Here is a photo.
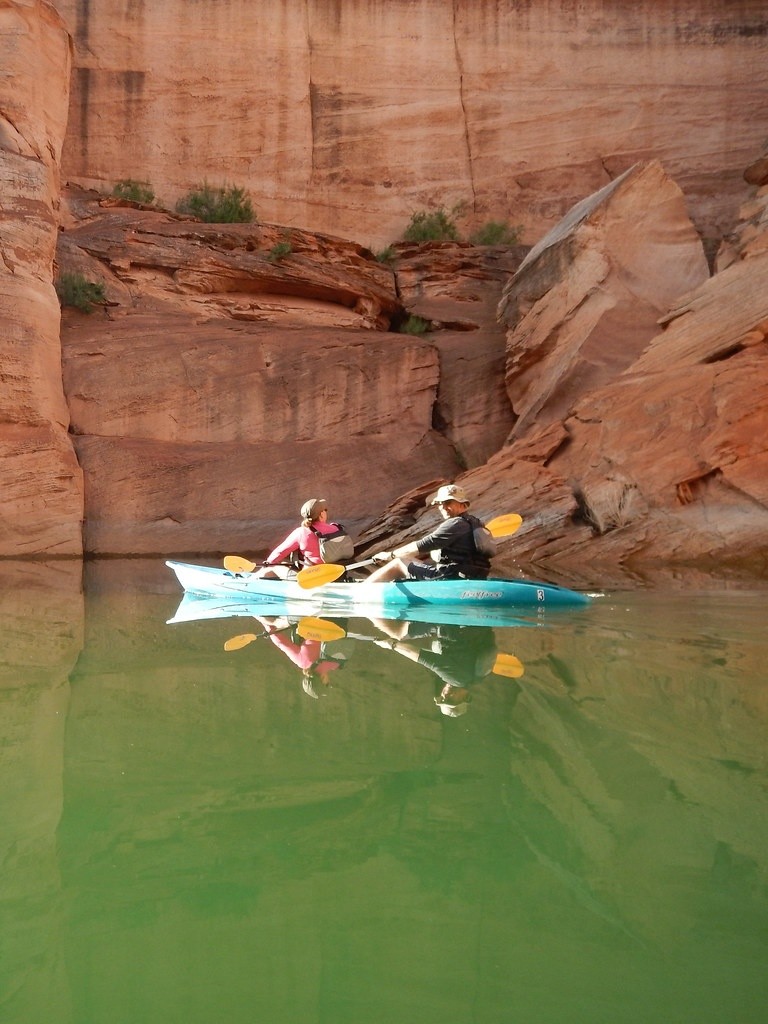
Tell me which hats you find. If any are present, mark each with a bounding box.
[301,499,328,521]
[433,696,469,717]
[302,675,327,699]
[431,485,470,507]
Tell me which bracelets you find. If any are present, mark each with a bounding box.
[391,550,395,559]
[391,641,399,653]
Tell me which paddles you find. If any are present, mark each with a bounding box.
[223,624,297,653]
[296,614,526,679]
[223,555,295,573]
[295,513,523,590]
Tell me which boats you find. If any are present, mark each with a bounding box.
[165,560,593,608]
[166,591,542,627]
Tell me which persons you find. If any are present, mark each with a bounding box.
[348,484,496,583]
[252,616,348,700]
[253,499,354,582]
[369,617,497,718]
